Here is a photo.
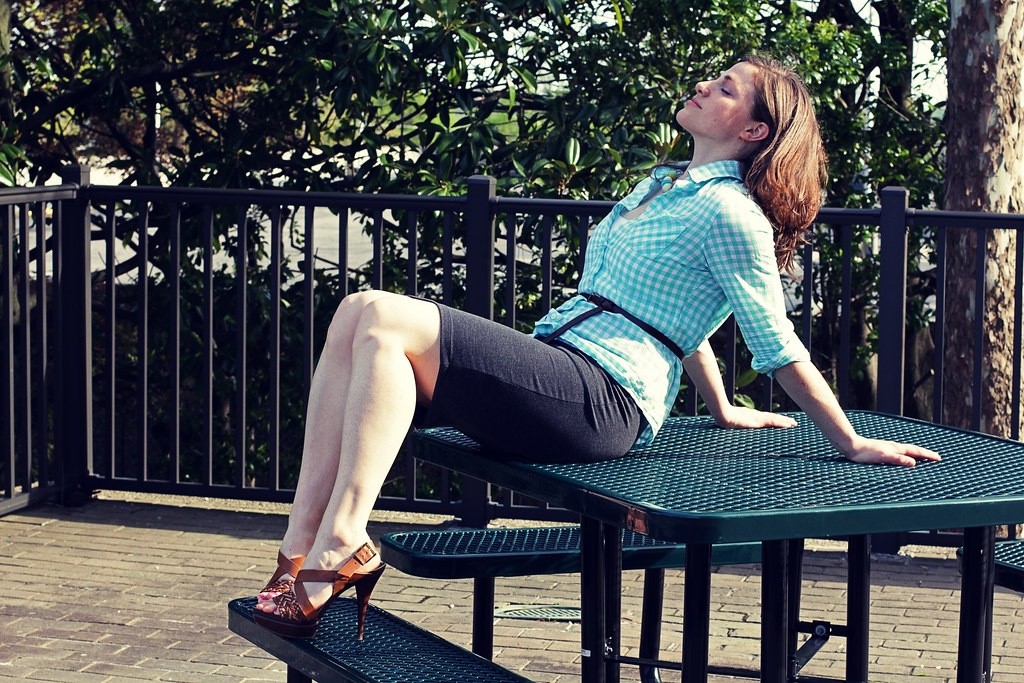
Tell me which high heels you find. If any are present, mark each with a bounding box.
[253,540,386,640]
[259,548,306,593]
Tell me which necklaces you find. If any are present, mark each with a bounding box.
[661,171,677,191]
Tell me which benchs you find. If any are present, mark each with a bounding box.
[227,596,533,683]
[956,540,1024,594]
[380,530,762,683]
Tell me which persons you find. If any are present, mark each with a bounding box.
[254,57,942,640]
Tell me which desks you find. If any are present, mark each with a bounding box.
[398,407,1024,683]
